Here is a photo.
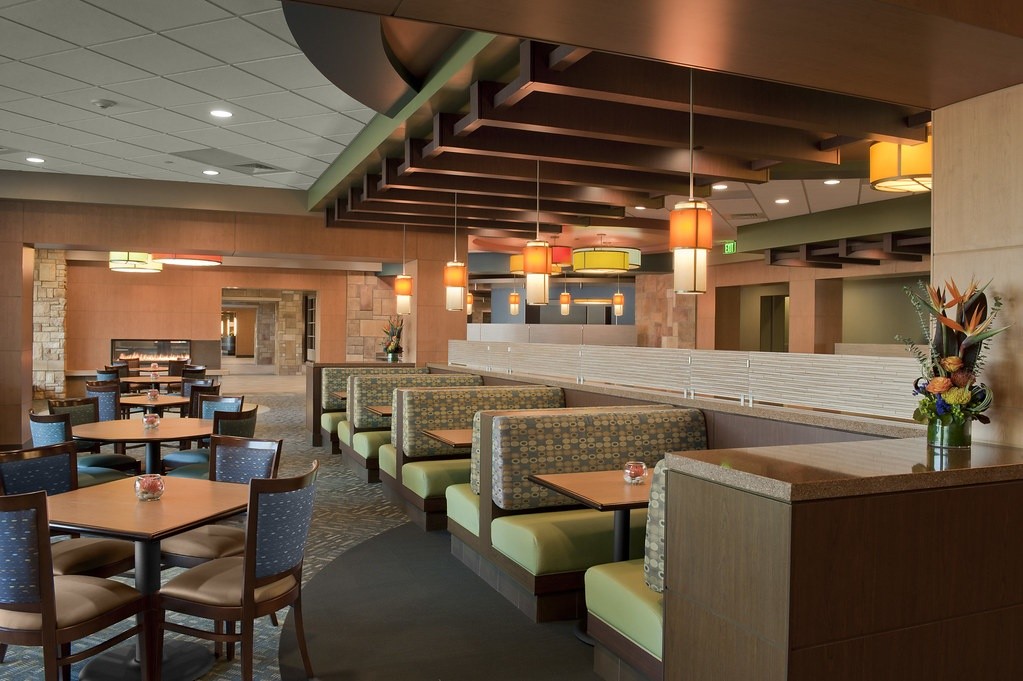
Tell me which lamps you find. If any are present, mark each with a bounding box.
[509,160,641,316]
[869,122,934,194]
[467,293,473,314]
[108,251,223,273]
[442,191,467,313]
[394,222,412,316]
[670,70,712,295]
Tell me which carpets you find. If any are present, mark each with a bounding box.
[278,520,654,681]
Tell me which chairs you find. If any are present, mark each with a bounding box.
[0,359,317,680]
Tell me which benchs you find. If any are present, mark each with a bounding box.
[319,366,711,681]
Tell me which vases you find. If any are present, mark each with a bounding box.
[387,350,399,363]
[924,410,971,448]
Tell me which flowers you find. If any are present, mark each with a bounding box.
[894,271,1015,422]
[379,316,404,351]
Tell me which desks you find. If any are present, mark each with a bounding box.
[420,428,474,448]
[364,406,393,417]
[119,374,184,392]
[128,365,167,377]
[47,474,258,681]
[70,418,214,477]
[118,394,193,417]
[329,390,346,401]
[527,467,655,647]
